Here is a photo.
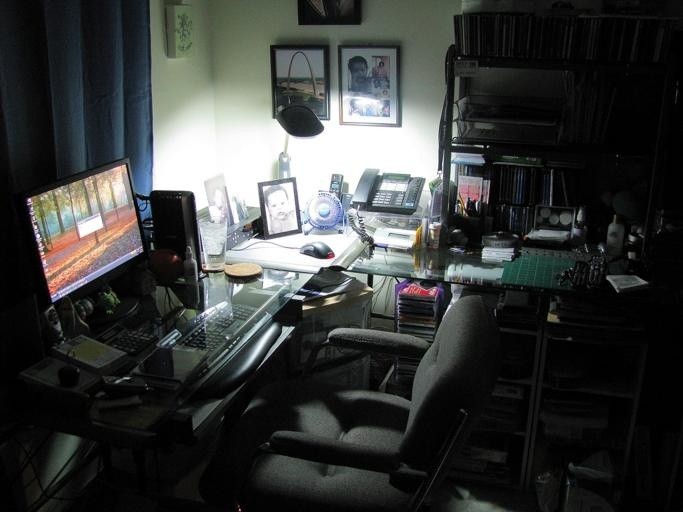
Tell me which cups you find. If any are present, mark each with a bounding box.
[198,215,228,272]
[627,232,645,261]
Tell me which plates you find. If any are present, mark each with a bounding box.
[224,262,262,277]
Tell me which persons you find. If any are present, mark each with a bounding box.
[262,184,297,234]
[346,56,372,95]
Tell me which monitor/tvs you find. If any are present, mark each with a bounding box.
[13,156,151,327]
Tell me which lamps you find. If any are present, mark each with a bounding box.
[276,105,323,178]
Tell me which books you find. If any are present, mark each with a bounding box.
[452,160,569,240]
[605,273,650,294]
[568,76,627,149]
[16,355,99,393]
[50,333,128,374]
[127,341,210,391]
[540,295,632,489]
[449,288,539,487]
[452,8,681,71]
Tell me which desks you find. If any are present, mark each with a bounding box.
[1,247,434,512]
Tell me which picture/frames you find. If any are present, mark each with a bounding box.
[257,178,305,240]
[268,43,406,132]
[298,0,363,26]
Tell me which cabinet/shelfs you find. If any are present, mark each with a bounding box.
[435,0,682,512]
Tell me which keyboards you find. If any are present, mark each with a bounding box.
[133,273,273,378]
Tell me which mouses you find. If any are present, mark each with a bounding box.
[299,239,336,259]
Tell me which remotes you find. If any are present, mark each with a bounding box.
[588,254,608,290]
[571,260,588,289]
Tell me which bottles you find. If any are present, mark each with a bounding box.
[573,207,588,247]
[606,213,626,255]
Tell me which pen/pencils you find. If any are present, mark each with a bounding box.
[454,192,483,217]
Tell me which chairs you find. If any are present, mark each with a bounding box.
[250,293,500,512]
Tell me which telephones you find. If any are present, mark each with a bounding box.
[350,168,425,215]
